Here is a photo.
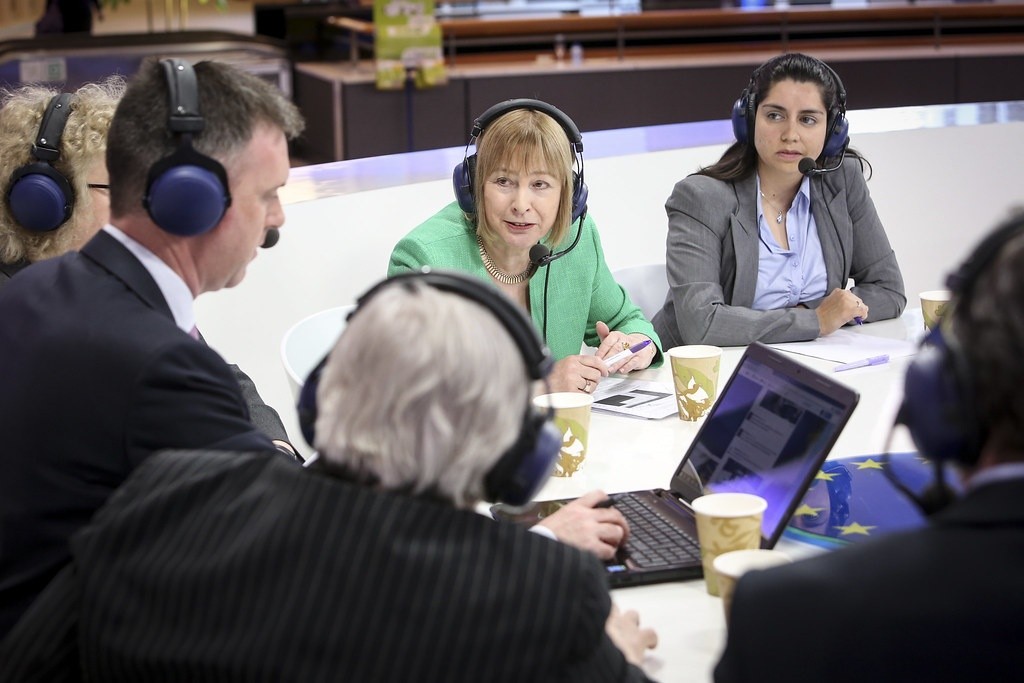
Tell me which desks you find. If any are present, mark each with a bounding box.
[474,307,959,683]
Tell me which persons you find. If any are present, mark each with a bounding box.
[0,57,629,629]
[0,269,665,683]
[0,76,128,284]
[649,52,909,350]
[386,98,665,398]
[715,212,1024,683]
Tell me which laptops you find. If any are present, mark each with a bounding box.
[489,341,859,590]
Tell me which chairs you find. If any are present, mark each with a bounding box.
[279,303,356,407]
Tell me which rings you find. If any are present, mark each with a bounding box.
[583,380,591,392]
[622,342,629,350]
[856,301,860,307]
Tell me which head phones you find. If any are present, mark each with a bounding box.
[4,94,76,235]
[732,55,850,158]
[295,269,560,509]
[903,219,1024,464]
[452,99,588,225]
[140,57,233,237]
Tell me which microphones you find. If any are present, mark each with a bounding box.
[530,217,585,266]
[798,151,845,176]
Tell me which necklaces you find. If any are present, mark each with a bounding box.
[760,191,789,223]
[474,225,534,284]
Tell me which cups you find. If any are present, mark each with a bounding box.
[920,291,951,339]
[692,494,768,597]
[714,549,790,630]
[667,345,721,424]
[532,393,593,477]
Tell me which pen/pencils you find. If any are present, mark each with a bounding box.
[855,317,862,325]
[603,339,652,369]
[831,354,890,372]
[594,489,630,510]
